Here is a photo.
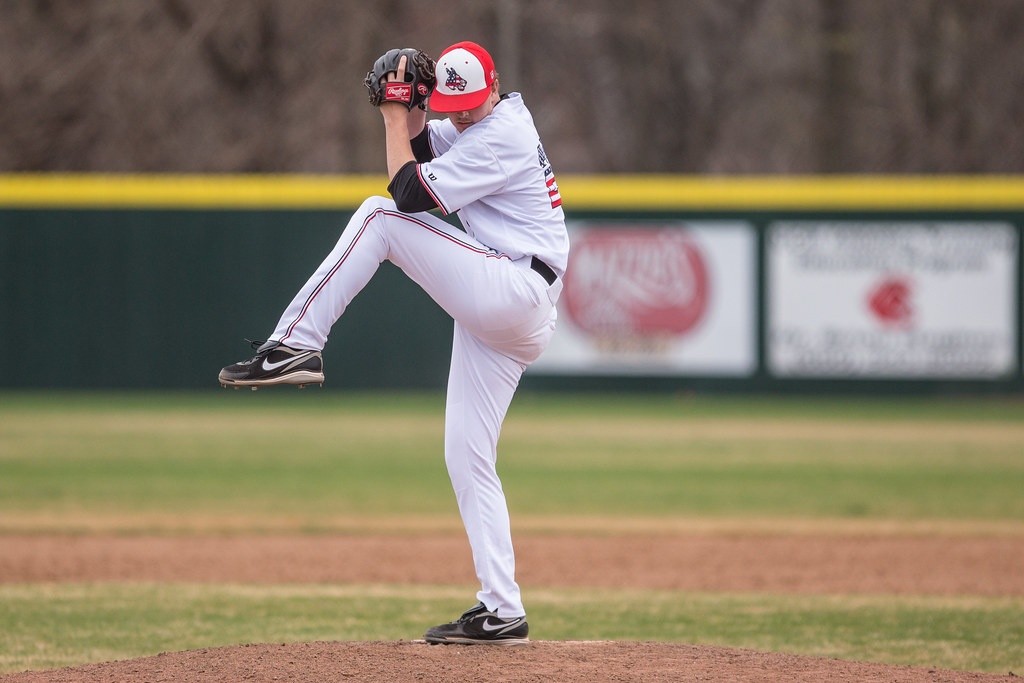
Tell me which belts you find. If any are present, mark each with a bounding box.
[530,256,557,286]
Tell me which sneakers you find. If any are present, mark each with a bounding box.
[218,338,324,391]
[423,602,529,645]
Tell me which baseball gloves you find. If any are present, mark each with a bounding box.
[365,48,436,113]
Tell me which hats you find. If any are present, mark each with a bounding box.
[429,41,496,112]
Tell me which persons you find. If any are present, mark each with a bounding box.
[218,41,569,645]
